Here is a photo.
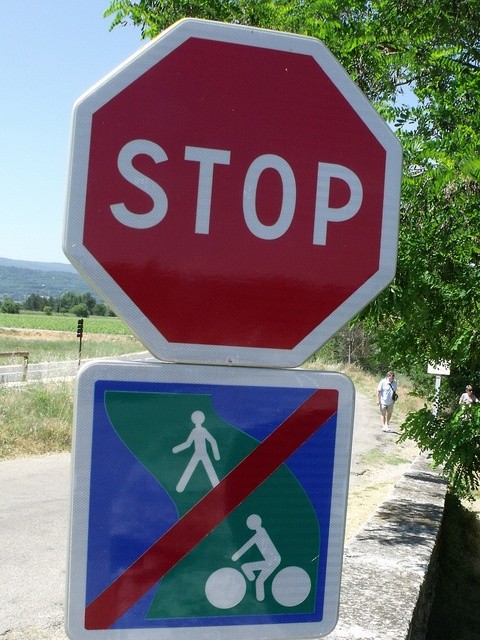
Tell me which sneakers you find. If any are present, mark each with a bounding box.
[382,426,386,431]
[386,425,391,430]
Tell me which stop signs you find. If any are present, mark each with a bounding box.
[65,362,355,639]
[63,17,404,369]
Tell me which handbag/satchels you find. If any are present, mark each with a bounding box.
[389,384,398,400]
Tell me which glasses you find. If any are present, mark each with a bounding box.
[467,389,473,392]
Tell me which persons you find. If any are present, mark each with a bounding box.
[375,370,399,433]
[458,384,479,419]
